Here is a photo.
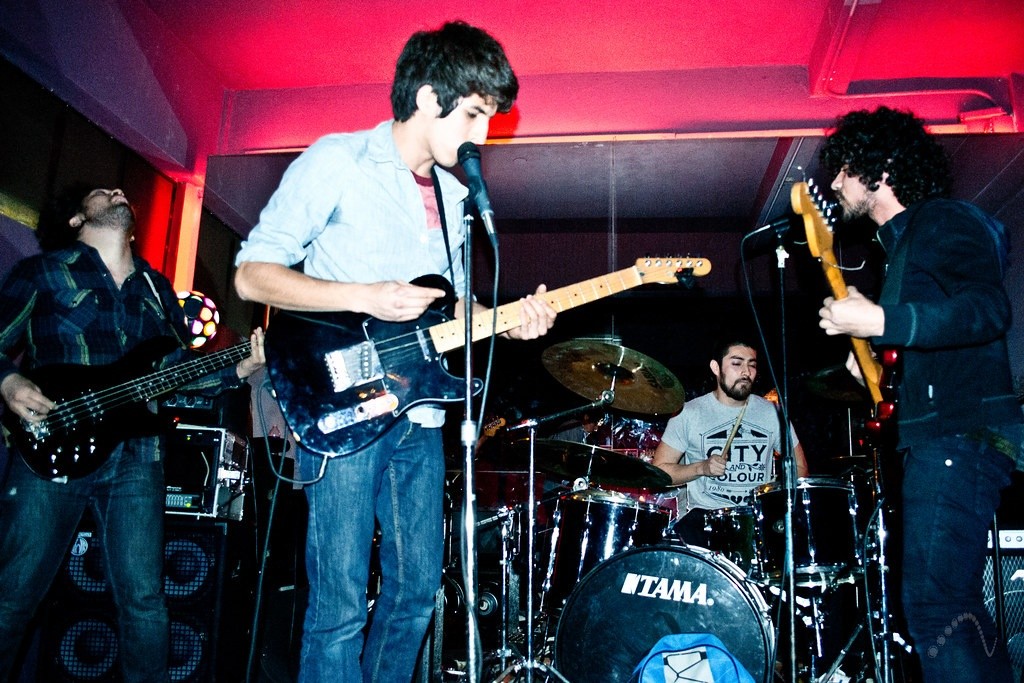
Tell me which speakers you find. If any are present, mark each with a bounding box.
[20,515,252,683]
[981,548,1024,683]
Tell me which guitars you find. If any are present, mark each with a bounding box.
[264,253,713,460]
[787,178,901,467]
[0,336,253,484]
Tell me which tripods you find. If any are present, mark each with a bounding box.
[822,461,921,683]
[476,376,615,683]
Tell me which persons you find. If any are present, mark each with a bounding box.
[0,178,266,683]
[234,20,558,683]
[818,106,1023,683]
[541,400,610,494]
[652,336,810,683]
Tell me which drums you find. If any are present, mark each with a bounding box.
[750,476,863,588]
[540,489,674,613]
[703,504,785,588]
[551,544,779,683]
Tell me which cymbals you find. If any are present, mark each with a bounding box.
[545,340,688,419]
[508,434,675,498]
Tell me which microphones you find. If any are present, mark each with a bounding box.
[458,141,499,248]
[744,201,843,239]
